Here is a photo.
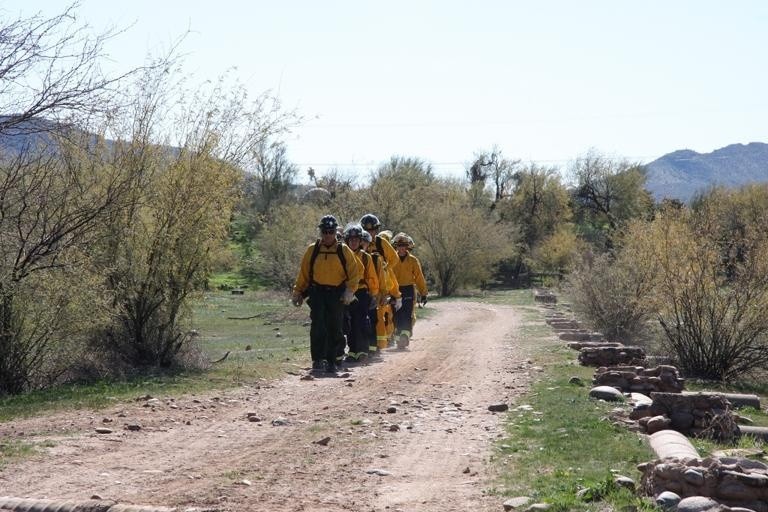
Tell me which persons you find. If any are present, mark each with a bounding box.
[292,213,428,375]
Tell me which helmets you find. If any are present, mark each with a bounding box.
[391,232,416,249]
[343,223,362,238]
[316,215,339,228]
[359,213,382,230]
[361,230,373,243]
[377,229,393,242]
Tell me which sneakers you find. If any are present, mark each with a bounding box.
[311,333,410,373]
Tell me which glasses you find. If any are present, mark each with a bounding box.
[321,229,336,235]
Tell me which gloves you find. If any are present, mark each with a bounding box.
[291,293,306,308]
[394,298,403,312]
[339,287,355,306]
[369,296,378,311]
[420,295,428,307]
